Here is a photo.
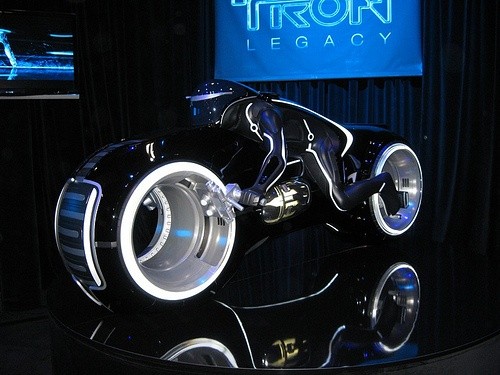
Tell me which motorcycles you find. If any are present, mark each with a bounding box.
[52,122,426,316]
[86,241,425,369]
